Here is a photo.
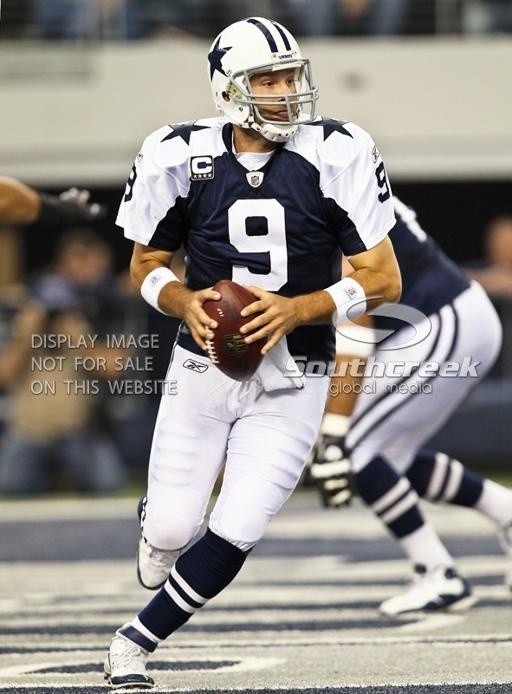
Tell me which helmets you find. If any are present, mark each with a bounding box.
[208,16,319,144]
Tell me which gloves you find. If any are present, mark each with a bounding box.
[310,432,355,509]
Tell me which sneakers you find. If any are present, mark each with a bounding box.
[102,627,156,689]
[136,496,183,591]
[500,521,512,593]
[377,562,479,622]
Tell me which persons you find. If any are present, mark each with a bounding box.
[308,194,511,621]
[454,202,511,381]
[103,17,403,688]
[0,174,182,499]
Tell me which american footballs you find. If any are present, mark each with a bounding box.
[202,280,268,382]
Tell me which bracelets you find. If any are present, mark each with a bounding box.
[323,276,367,326]
[139,266,181,316]
